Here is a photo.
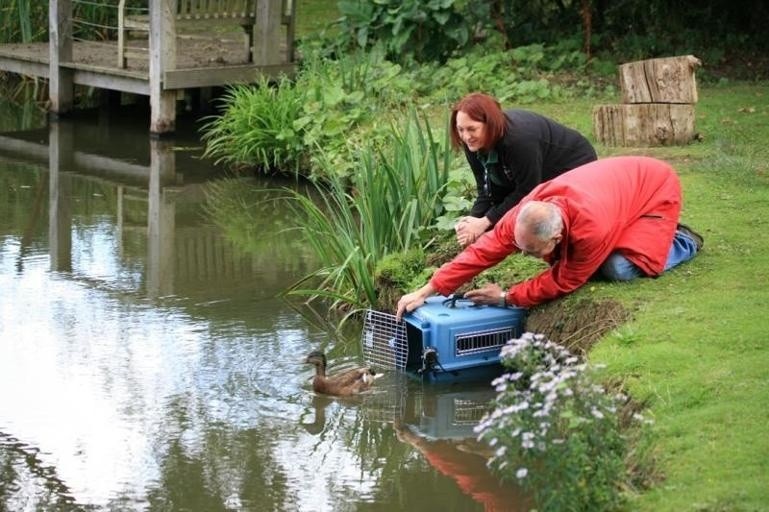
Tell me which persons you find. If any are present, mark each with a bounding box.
[449,93,598,248]
[396,156,705,325]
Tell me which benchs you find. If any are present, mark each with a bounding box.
[118,0,296,68]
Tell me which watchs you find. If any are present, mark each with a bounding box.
[497,289,507,309]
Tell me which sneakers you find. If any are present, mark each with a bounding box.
[675,222,704,250]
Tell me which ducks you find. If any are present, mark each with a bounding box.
[299,350,388,396]
